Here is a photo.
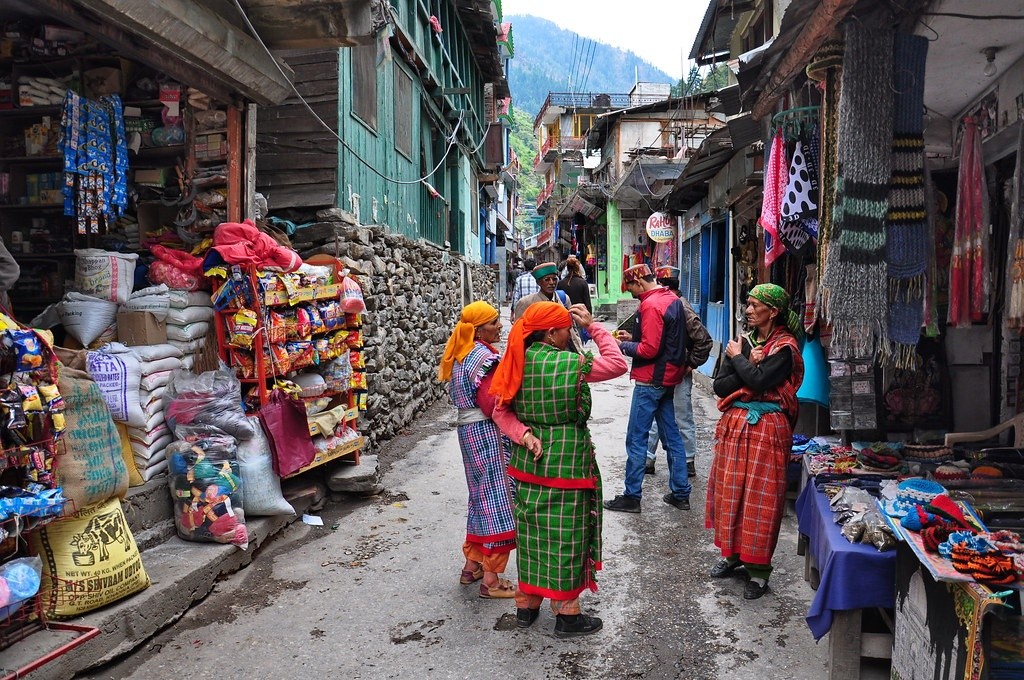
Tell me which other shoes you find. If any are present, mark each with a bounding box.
[711,559,744,577]
[744,579,768,599]
[686,461,696,477]
[645,460,655,474]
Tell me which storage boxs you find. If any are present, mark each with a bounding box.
[136,168,168,187]
[125,116,156,132]
[116,312,168,346]
[81,55,134,102]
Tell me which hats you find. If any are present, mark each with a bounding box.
[654,266,680,279]
[624,264,653,283]
[531,262,557,281]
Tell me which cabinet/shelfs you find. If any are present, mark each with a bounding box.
[0,48,239,318]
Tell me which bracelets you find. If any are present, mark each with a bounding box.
[523,431,531,439]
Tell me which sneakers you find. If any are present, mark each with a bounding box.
[663,493,690,510]
[604,494,641,513]
[517,608,539,627]
[479,577,517,598]
[460,563,484,584]
[554,613,602,637]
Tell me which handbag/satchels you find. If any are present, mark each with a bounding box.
[257,388,315,477]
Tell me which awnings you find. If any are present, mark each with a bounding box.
[664,114,761,216]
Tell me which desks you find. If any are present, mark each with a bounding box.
[794,452,898,680]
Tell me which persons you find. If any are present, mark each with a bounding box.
[513,262,579,339]
[557,253,592,316]
[703,283,805,599]
[486,301,630,638]
[436,300,520,600]
[510,258,542,325]
[599,264,691,512]
[0,236,21,311]
[644,264,713,476]
